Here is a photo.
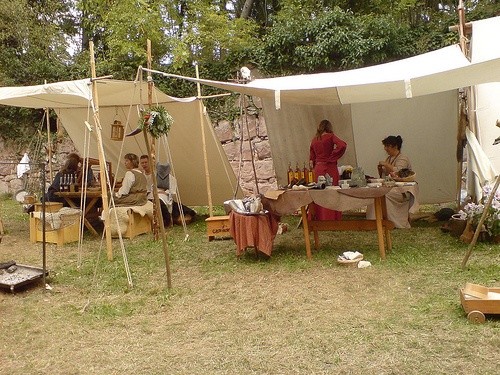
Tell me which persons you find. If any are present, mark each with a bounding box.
[139,154,177,229]
[307,120,347,221]
[99,153,147,225]
[47,153,98,222]
[367,135,417,229]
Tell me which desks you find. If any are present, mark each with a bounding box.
[54,188,119,237]
[263,180,417,260]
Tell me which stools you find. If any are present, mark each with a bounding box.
[30,201,411,248]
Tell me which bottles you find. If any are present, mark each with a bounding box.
[243,187,255,213]
[343,167,352,179]
[287,160,314,185]
[60,174,79,192]
[254,193,263,213]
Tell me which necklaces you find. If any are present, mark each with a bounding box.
[389,153,400,164]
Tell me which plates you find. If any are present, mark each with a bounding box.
[88,187,100,191]
[394,181,417,187]
[368,178,386,183]
[327,185,342,190]
[368,183,383,188]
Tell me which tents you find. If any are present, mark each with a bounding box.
[138,15,500,269]
[0,70,247,293]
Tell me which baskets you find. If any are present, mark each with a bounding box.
[391,168,416,182]
